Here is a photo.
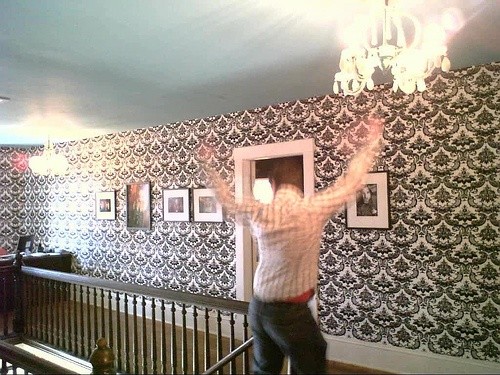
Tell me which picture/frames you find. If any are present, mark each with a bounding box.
[125,181,151,231]
[192,188,224,223]
[162,187,190,222]
[345,170,391,230]
[95,191,116,220]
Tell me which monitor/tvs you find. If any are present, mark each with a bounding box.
[17,235,35,255]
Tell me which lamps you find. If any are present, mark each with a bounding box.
[333,0,451,96]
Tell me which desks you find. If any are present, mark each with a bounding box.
[0,253,71,312]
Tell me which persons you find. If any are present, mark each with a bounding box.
[355,184,378,216]
[100,199,110,212]
[193,116,384,375]
